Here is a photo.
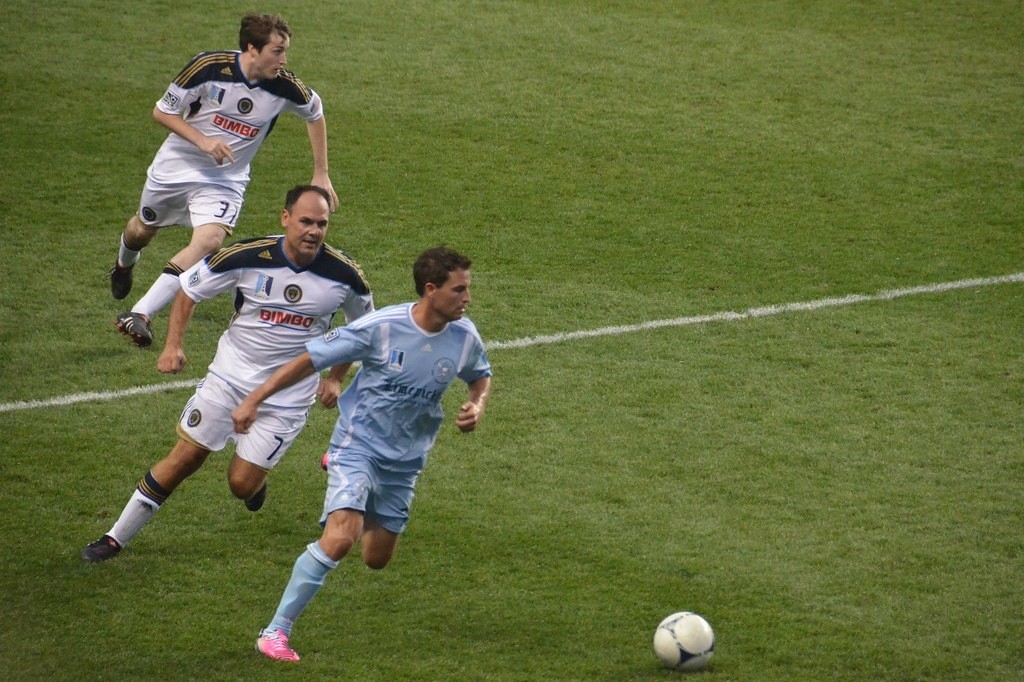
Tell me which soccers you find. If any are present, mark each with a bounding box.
[653,612,715,672]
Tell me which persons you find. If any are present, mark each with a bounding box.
[108,14,340,349]
[228,249,494,662]
[79,184,378,561]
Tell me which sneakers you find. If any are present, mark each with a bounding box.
[255,628,300,663]
[245,480,267,511]
[322,451,327,471]
[109,255,135,299]
[81,535,121,562]
[113,312,153,348]
